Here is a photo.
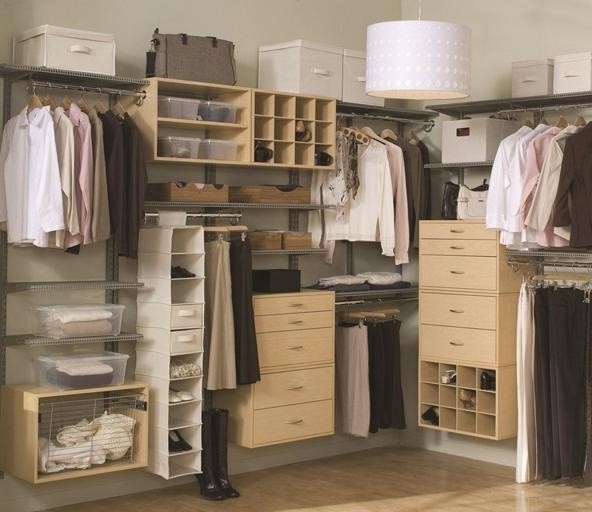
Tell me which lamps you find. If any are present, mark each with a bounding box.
[365,0,470,101]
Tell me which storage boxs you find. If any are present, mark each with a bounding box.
[157,134,203,160]
[510,59,553,96]
[157,95,202,121]
[32,349,129,392]
[24,303,125,339]
[198,139,240,162]
[198,100,240,123]
[256,39,342,102]
[10,24,115,77]
[440,118,522,164]
[342,48,384,108]
[552,53,592,97]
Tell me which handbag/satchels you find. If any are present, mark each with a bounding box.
[455,184,489,220]
[145,50,156,78]
[441,181,459,220]
[152,32,239,86]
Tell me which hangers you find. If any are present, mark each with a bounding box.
[333,298,400,320]
[521,103,586,129]
[519,261,592,303]
[222,213,250,243]
[21,81,127,119]
[198,217,228,243]
[336,111,422,148]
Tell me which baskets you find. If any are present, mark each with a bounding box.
[37,391,139,477]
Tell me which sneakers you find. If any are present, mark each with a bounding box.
[171,267,196,278]
[169,389,181,402]
[174,389,193,401]
[420,405,440,427]
[441,368,456,384]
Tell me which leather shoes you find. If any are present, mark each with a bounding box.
[481,371,496,392]
[172,429,192,450]
[170,434,182,451]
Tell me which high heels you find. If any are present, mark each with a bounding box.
[459,388,476,409]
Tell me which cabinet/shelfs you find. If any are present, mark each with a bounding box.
[251,88,337,171]
[139,201,328,257]
[3,280,144,352]
[419,219,538,367]
[210,288,337,451]
[416,359,518,440]
[0,379,150,485]
[135,225,203,481]
[115,77,252,167]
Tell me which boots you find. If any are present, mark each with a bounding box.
[209,407,240,497]
[195,412,226,500]
